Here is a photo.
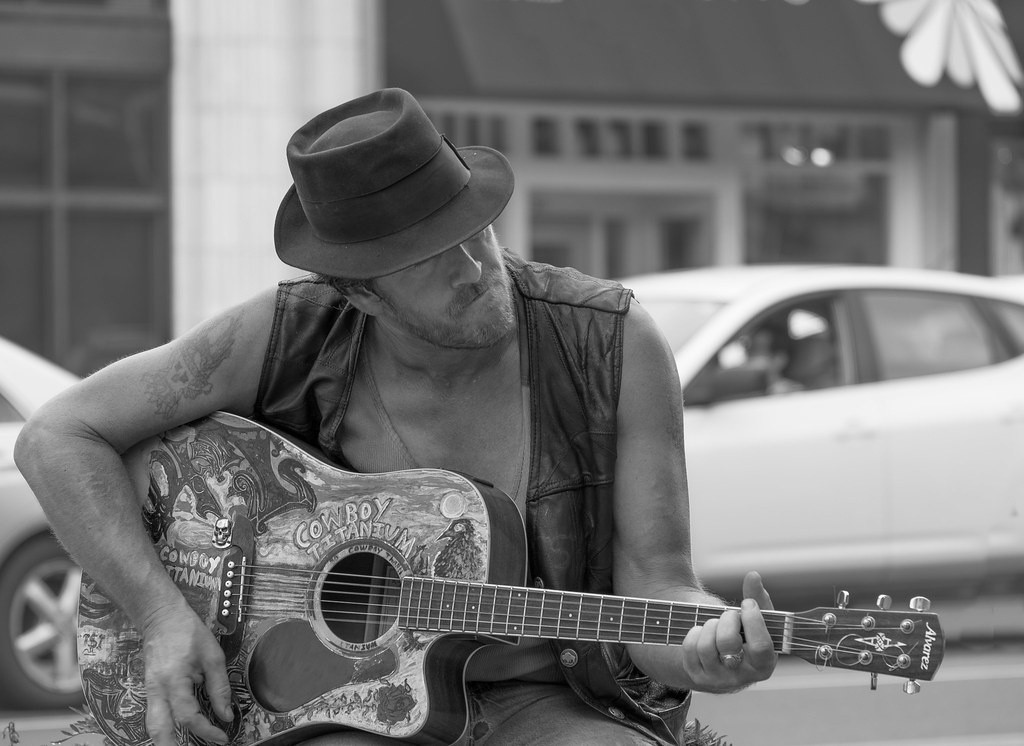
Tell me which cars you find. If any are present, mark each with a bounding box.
[582,260,1023,644]
[0,332,125,716]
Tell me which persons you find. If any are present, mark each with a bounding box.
[745,320,807,397]
[12,84,780,746]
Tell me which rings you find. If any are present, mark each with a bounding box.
[717,647,746,672]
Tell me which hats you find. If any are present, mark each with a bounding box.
[274,86,515,281]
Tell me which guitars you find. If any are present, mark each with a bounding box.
[74,409,947,746]
